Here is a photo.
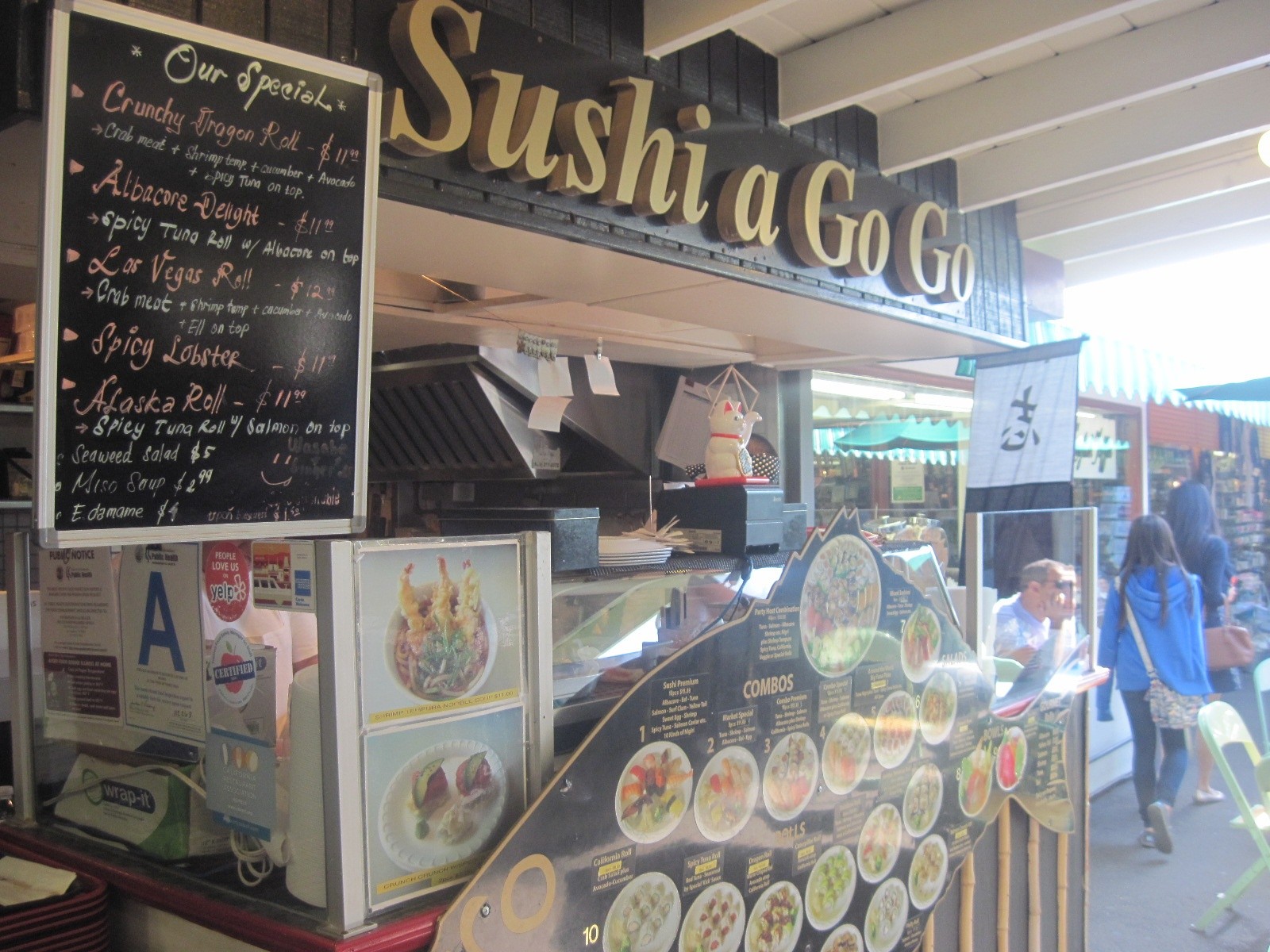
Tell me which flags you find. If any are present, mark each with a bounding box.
[966,339,1080,513]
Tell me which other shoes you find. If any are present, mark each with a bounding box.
[1148,802,1174,854]
[1195,788,1223,804]
[1143,828,1154,849]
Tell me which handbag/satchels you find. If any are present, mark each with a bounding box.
[1199,593,1255,669]
[1150,680,1205,729]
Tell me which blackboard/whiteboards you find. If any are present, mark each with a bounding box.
[31,0,383,553]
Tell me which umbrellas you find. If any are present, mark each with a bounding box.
[1173,377,1270,402]
[833,415,1130,458]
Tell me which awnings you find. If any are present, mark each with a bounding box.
[812,428,959,467]
[956,321,1270,426]
[811,372,1103,438]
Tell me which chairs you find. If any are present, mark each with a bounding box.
[1189,658,1270,931]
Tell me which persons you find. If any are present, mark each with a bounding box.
[1096,516,1214,854]
[996,515,1053,598]
[1164,480,1238,802]
[986,558,1066,665]
[1076,523,1119,578]
[1042,564,1076,660]
[203,536,318,718]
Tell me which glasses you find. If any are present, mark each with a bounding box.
[1042,579,1064,589]
